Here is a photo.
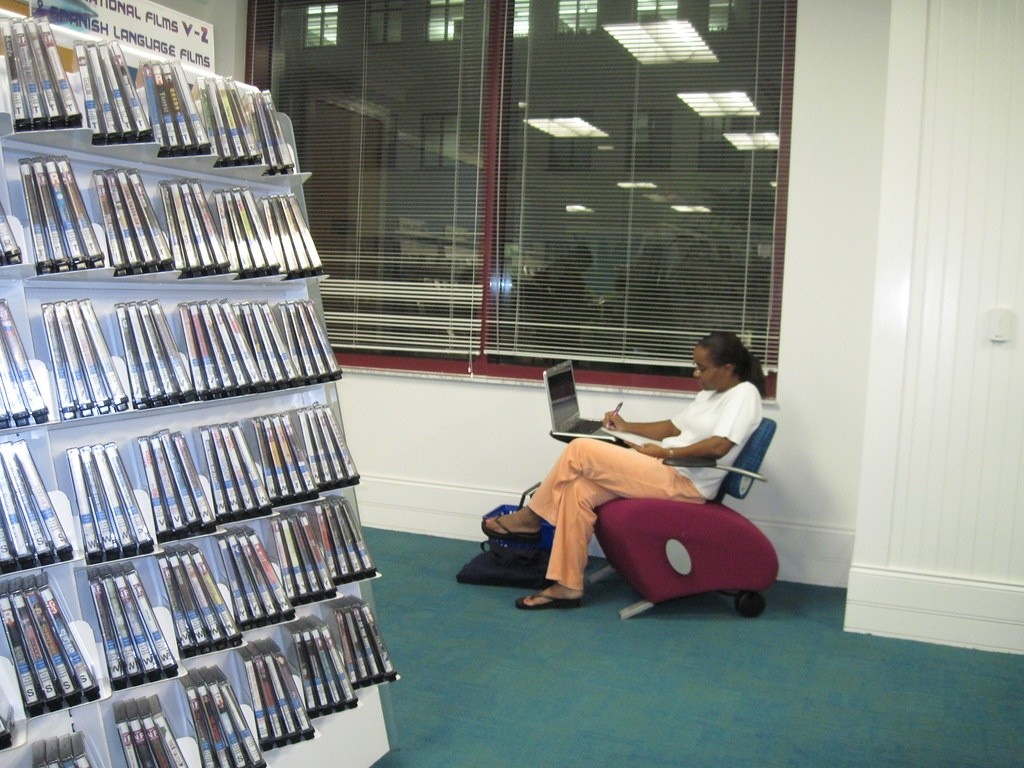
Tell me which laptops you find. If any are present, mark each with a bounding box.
[543,360,619,443]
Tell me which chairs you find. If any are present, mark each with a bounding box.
[582,417,779,624]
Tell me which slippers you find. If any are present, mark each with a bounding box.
[515,594,580,609]
[482,515,541,544]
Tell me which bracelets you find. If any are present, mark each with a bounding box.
[669,450,674,458]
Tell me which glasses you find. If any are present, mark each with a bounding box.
[692,360,724,374]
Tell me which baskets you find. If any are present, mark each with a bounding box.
[483,481,556,550]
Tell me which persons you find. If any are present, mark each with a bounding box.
[482,330,764,610]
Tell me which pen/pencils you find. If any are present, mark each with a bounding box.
[606,400,623,429]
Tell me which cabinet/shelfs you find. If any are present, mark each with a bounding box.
[0,11,393,768]
